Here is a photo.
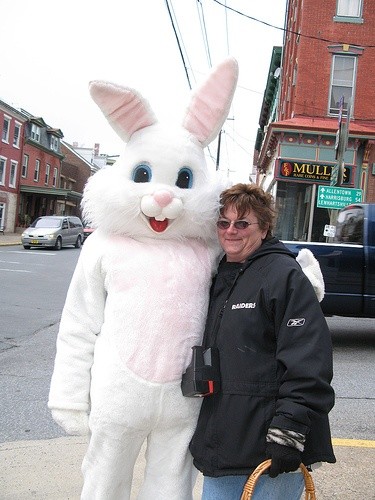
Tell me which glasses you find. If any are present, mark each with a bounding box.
[216,217,259,230]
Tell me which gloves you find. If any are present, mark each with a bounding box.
[51,409,89,436]
[266,441,301,478]
[296,248,325,303]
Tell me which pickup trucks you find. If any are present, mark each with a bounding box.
[271,205,375,320]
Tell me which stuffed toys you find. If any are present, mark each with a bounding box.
[48,56,325,500]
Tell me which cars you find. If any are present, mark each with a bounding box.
[20,215,84,251]
[83,224,94,241]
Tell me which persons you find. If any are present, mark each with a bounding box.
[189,183,336,500]
